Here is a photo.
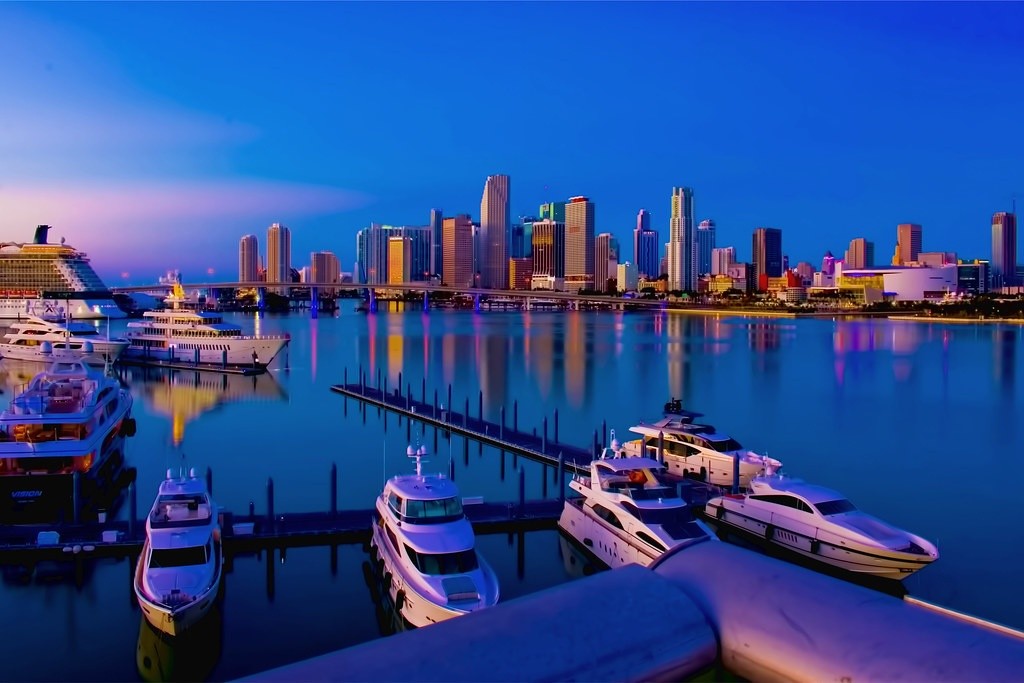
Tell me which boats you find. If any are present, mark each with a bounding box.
[370,436,500,631]
[0,291,40,299]
[133,464,225,640]
[137,597,224,683]
[1,295,132,365]
[557,428,720,569]
[704,450,939,583]
[620,397,784,492]
[0,300,136,525]
[120,276,292,369]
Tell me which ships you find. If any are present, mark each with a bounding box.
[0,225,128,320]
